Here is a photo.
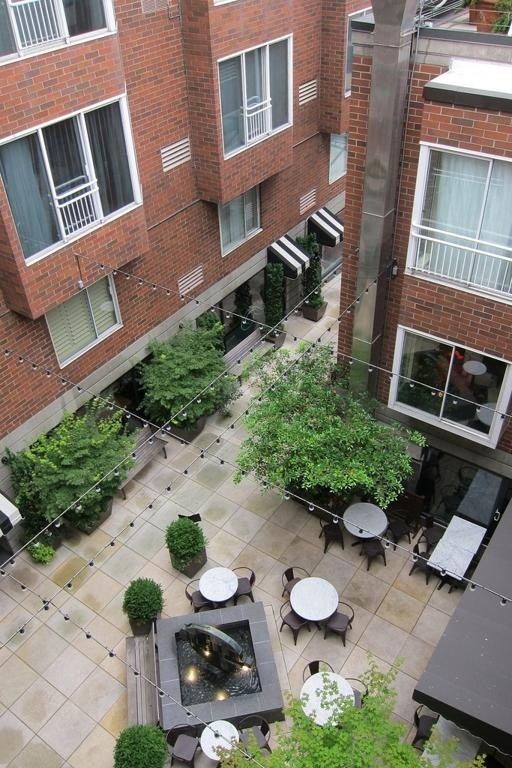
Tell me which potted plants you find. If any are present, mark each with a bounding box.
[260,262,288,348]
[138,348,227,444]
[14,394,136,535]
[166,516,209,578]
[120,578,164,638]
[298,232,329,322]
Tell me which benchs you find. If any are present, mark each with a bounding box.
[217,327,274,386]
[107,424,168,500]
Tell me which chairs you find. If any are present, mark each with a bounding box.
[183,567,256,611]
[301,659,369,719]
[318,508,412,570]
[279,567,355,647]
[163,715,271,768]
[409,466,505,592]
[411,705,440,751]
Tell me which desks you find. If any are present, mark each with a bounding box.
[298,672,355,729]
[344,502,389,546]
[420,715,486,768]
